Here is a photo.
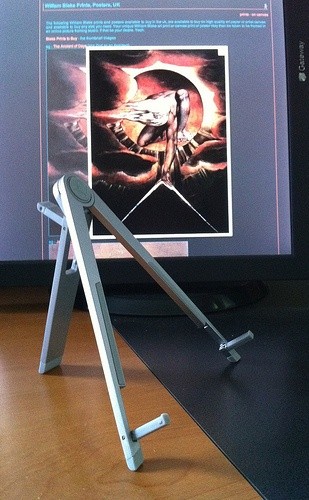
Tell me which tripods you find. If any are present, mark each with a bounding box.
[24,170,255,472]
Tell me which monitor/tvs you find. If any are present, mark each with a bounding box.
[0,1,308,287]
